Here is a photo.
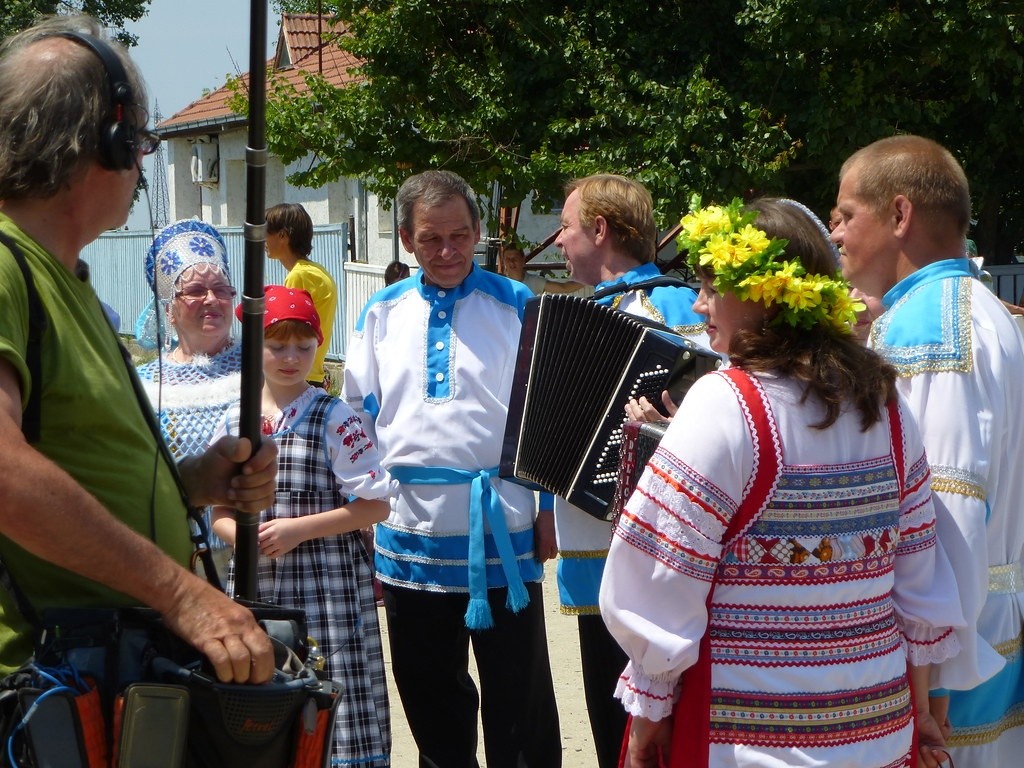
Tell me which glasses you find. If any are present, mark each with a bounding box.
[130,125,164,155]
[171,286,237,301]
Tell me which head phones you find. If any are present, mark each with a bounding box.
[56,31,138,169]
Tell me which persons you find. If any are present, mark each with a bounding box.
[338,170,731,768]
[384,260,411,287]
[0,10,393,767]
[504,242,585,296]
[599,133,1024,768]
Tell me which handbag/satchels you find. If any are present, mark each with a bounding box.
[1,598,344,768]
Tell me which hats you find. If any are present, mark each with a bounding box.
[142,219,231,350]
